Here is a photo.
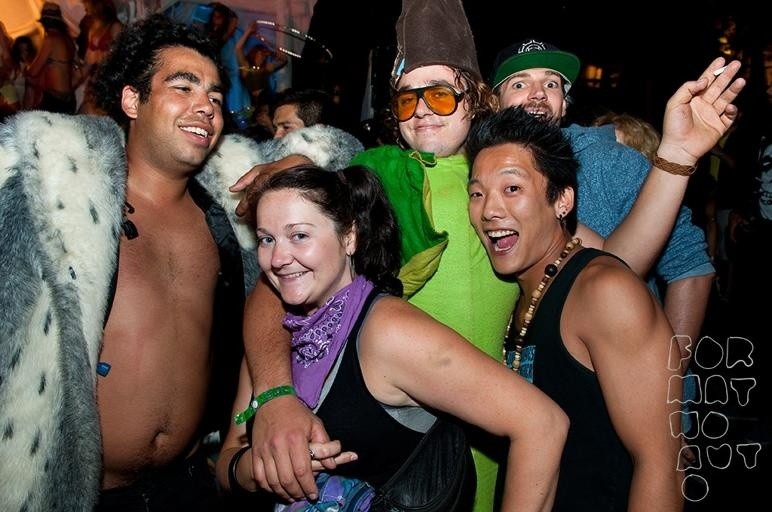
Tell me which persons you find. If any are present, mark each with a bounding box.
[0,0,772,511]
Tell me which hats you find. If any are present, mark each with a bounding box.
[37,2,64,23]
[492,38,582,99]
[391,0,481,81]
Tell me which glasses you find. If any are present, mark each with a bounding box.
[388,83,470,123]
